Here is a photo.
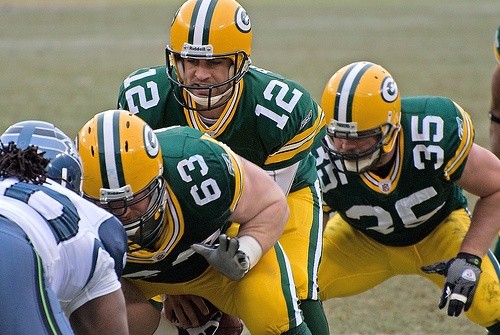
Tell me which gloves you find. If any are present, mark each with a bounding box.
[190,234,250,281]
[420,252,482,317]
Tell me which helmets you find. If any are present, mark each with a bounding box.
[73,110,163,202]
[0,120,84,198]
[321,61,402,152]
[169,0,252,71]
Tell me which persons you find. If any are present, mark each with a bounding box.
[72,110,311,335]
[0,120,131,335]
[317,60,500,335]
[115,0,330,335]
[489,28,500,158]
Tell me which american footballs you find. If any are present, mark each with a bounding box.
[171,297,243,335]
